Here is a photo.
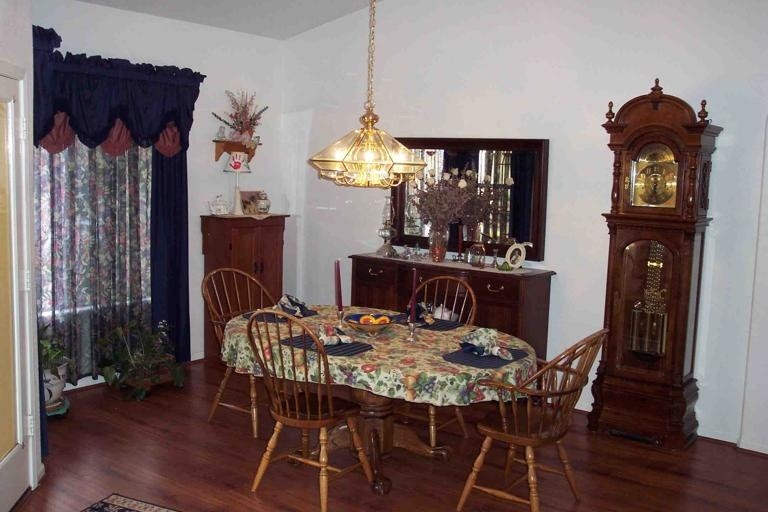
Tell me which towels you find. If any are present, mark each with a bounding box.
[459,326,515,362]
[434,302,459,322]
[311,321,354,351]
[271,293,307,318]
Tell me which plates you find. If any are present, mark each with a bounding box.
[343,314,399,337]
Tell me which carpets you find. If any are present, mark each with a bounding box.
[76,489,181,512]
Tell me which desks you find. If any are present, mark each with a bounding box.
[221,304,538,466]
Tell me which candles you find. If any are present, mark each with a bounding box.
[335,260,343,311]
[411,270,417,322]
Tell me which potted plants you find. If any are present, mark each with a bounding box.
[94,319,187,401]
[38,324,74,418]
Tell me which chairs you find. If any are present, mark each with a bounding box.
[201,268,277,439]
[247,309,374,511]
[406,276,477,447]
[455,326,610,512]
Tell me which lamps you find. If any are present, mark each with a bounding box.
[223,152,251,215]
[307,1,427,189]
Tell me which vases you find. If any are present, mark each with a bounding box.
[467,226,486,267]
[212,140,262,163]
[427,225,449,263]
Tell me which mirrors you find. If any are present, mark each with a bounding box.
[389,137,550,262]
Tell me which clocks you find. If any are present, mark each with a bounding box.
[587,79,723,453]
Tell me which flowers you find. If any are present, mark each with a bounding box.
[211,89,268,140]
[451,167,514,228]
[409,168,467,231]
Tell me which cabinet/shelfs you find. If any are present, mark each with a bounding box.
[200,215,291,347]
[347,251,556,408]
